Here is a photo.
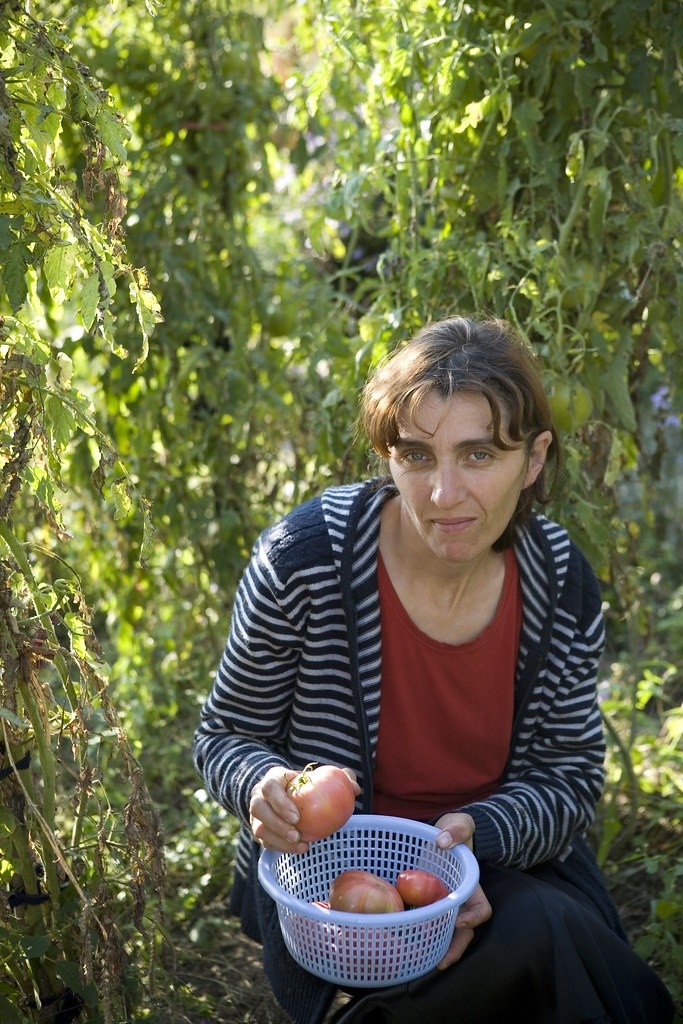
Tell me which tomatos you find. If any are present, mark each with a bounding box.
[293,869,450,968]
[283,763,354,842]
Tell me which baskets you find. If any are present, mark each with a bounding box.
[256,815,480,988]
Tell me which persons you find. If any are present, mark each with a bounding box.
[192,313,683,1024]
